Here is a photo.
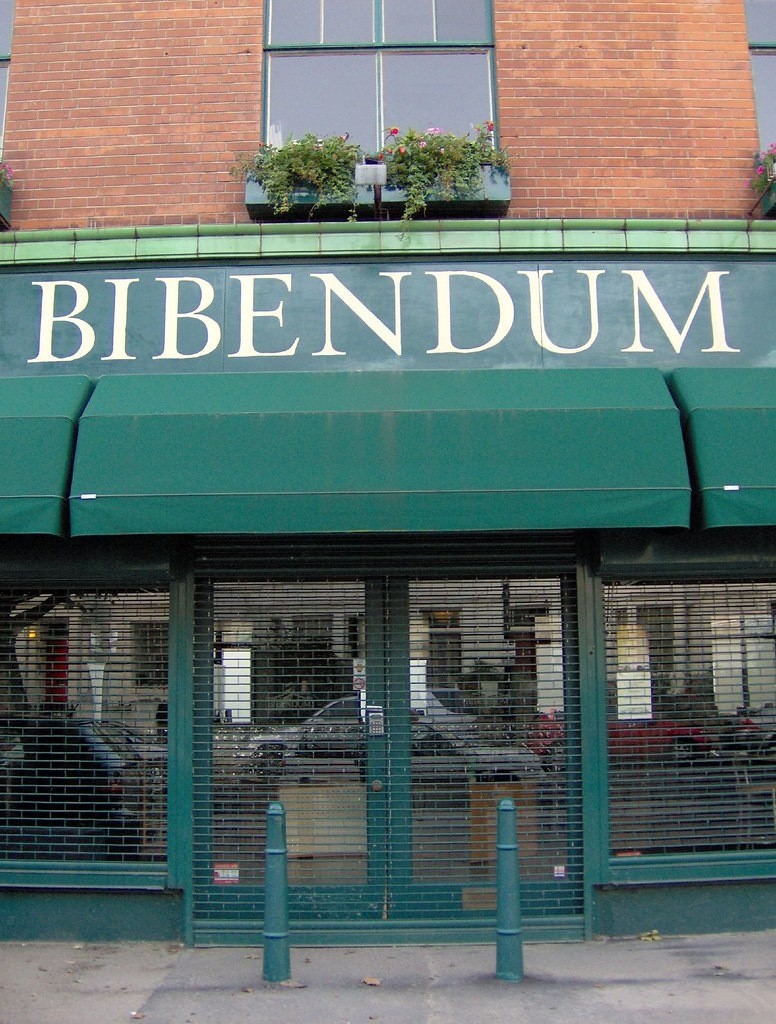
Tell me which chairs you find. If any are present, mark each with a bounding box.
[732,752,776,849]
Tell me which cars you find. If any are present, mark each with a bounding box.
[237,686,460,782]
[527,694,720,776]
[279,707,547,807]
[86,720,243,821]
[724,705,776,772]
[0,716,160,861]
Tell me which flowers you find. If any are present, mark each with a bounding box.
[229,127,365,227]
[0,159,15,192]
[749,142,776,191]
[369,118,511,224]
